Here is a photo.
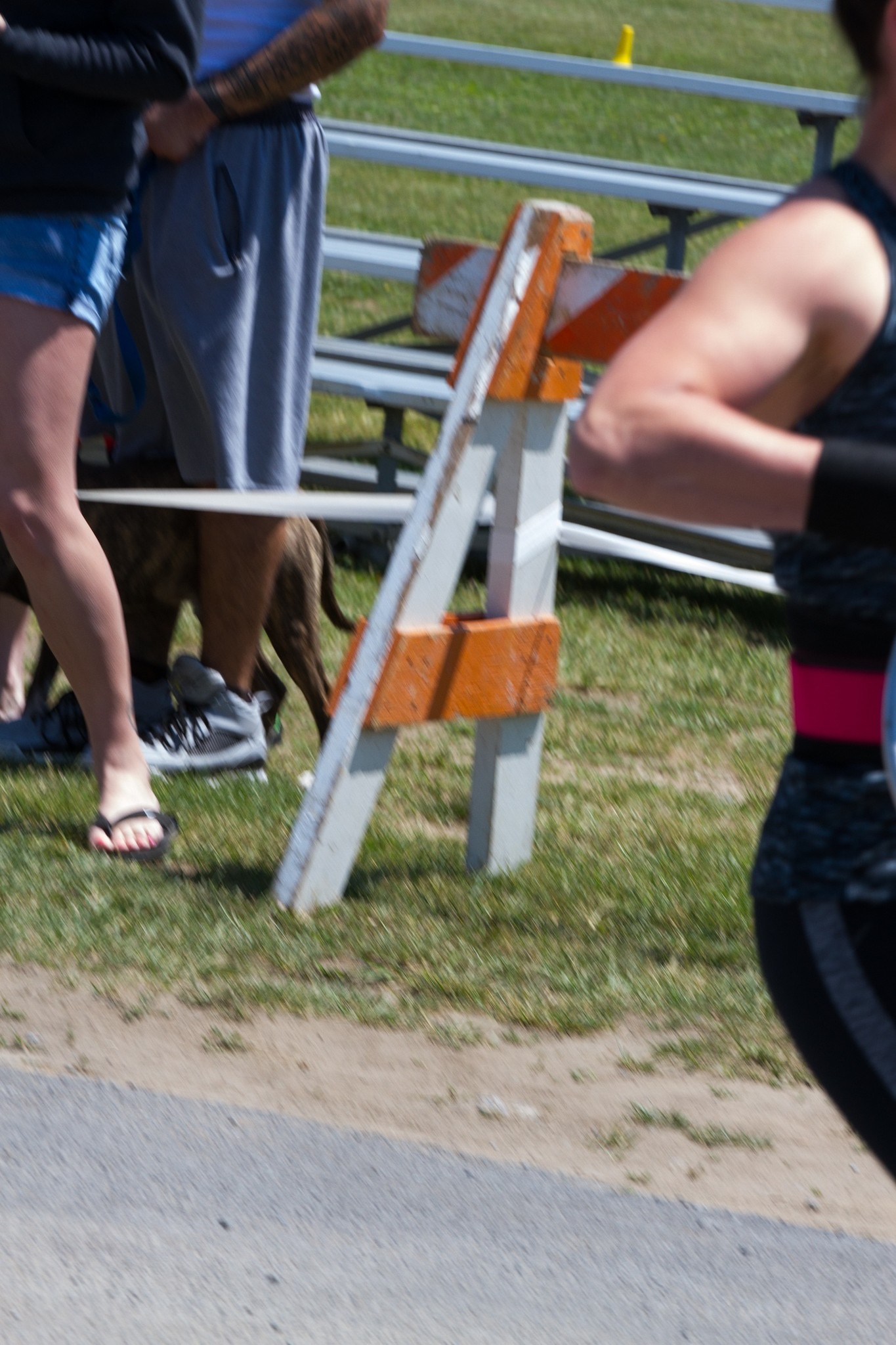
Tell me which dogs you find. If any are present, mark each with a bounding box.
[0,463,357,757]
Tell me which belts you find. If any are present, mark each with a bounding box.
[791,659,885,741]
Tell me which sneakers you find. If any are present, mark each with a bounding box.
[75,653,269,771]
[1,675,177,771]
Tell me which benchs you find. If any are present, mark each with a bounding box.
[297,29,865,599]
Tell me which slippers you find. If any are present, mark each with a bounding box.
[84,806,179,860]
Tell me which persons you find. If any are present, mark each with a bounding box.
[565,0,896,1182]
[0,1,388,866]
